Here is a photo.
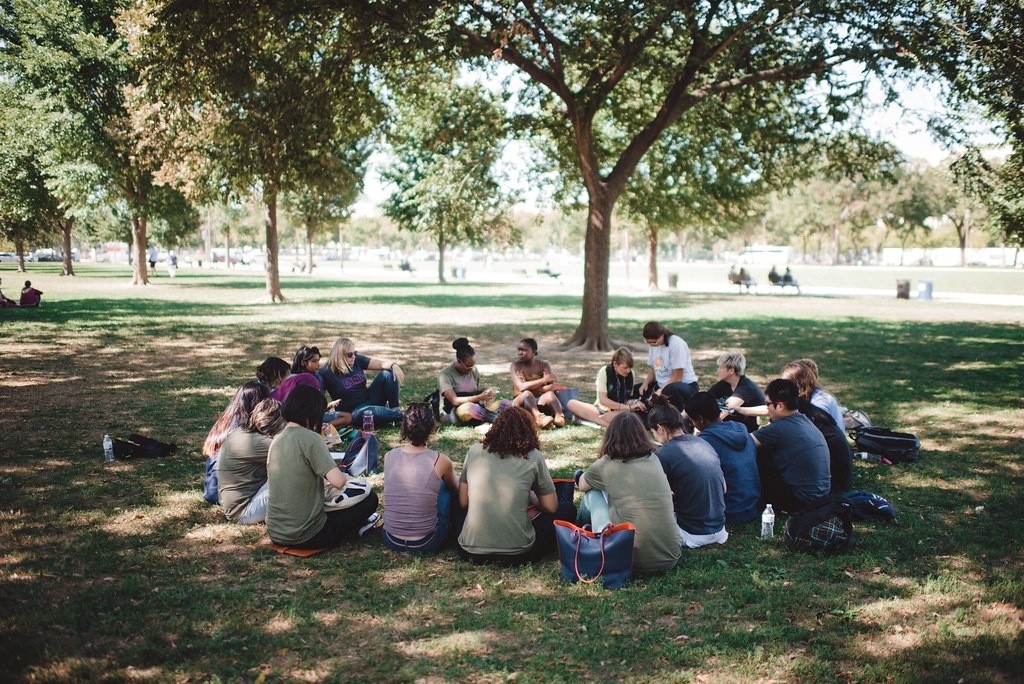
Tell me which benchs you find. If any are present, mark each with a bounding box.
[727,275,758,294]
[768,276,800,295]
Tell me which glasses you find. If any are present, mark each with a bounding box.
[765,400,784,407]
[343,351,357,358]
[644,338,660,344]
[406,402,430,411]
[460,361,475,369]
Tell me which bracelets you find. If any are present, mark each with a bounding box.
[628,404,631,411]
[391,362,398,369]
[633,400,638,404]
[619,403,623,410]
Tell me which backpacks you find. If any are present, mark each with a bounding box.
[784,495,853,554]
[849,426,920,462]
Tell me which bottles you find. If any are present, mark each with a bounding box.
[854,452,882,462]
[103,434,115,461]
[363,410,374,432]
[761,504,775,538]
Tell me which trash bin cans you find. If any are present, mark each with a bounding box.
[897,281,910,299]
[918,280,932,300]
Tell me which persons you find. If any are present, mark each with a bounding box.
[168,249,178,280]
[0,278,43,307]
[201,321,855,575]
[728,265,796,293]
[150,244,158,277]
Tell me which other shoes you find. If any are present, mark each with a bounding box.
[475,423,492,435]
[359,512,380,537]
[535,413,554,428]
[554,413,565,427]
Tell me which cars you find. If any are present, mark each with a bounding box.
[38,248,63,262]
[62,251,80,262]
[0,252,34,262]
[217,255,236,264]
[96,249,129,263]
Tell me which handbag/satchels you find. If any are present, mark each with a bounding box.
[840,409,872,430]
[553,519,635,588]
[552,478,576,502]
[337,429,377,477]
[323,479,372,513]
[336,426,363,441]
[111,433,178,460]
[423,388,440,423]
[838,490,896,522]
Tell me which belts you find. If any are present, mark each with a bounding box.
[385,531,436,547]
